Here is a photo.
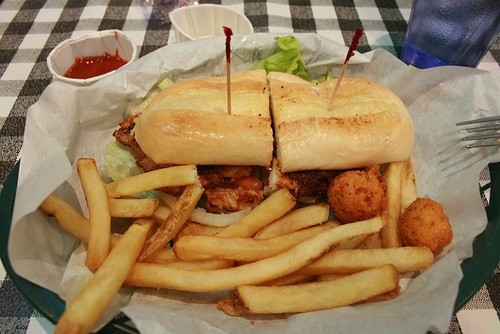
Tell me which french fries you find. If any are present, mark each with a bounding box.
[39,157,433,334]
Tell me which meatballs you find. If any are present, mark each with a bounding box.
[327,162,385,223]
[400,197,453,255]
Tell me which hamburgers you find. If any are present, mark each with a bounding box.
[104,34,417,214]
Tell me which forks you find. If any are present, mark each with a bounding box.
[455,115,500,148]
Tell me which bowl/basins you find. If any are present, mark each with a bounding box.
[169,3,253,42]
[47,30,137,86]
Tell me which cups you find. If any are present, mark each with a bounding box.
[401,0,499,68]
